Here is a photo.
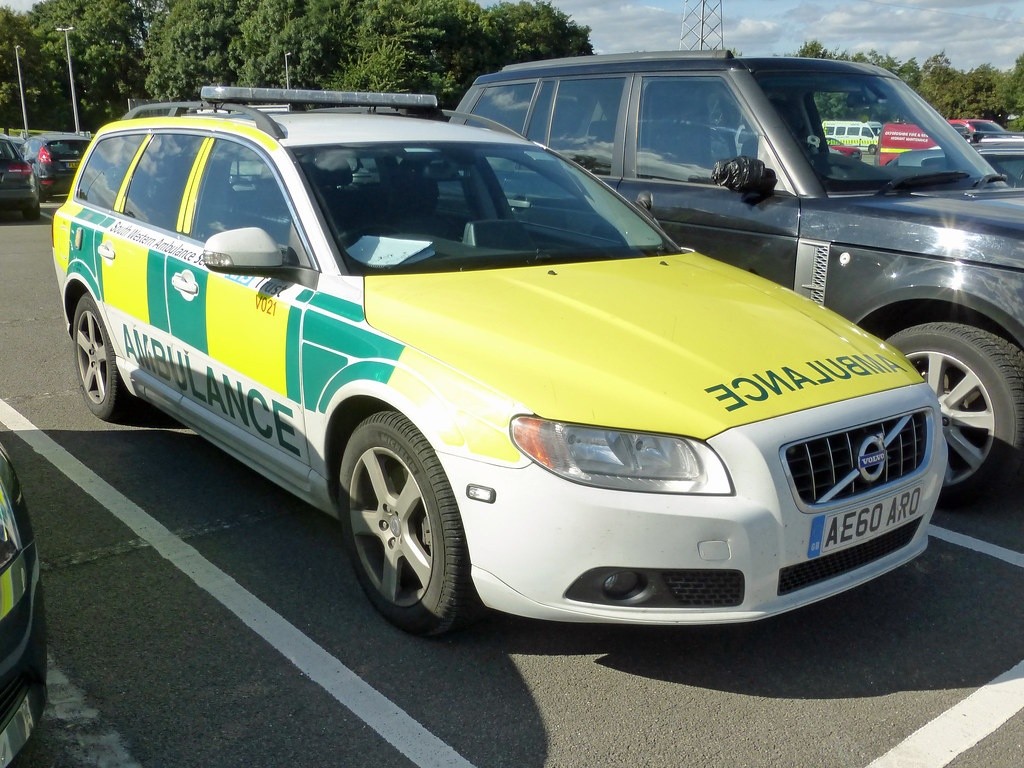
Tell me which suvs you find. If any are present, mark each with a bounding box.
[431,50,1024,508]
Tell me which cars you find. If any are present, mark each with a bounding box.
[825,137,862,161]
[0,446,49,768]
[885,132,1024,188]
[0,135,92,222]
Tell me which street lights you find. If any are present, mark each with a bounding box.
[57,25,79,136]
[285,53,291,89]
[15,46,29,140]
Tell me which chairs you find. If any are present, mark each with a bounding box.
[173,156,237,229]
[388,170,457,243]
[313,156,363,228]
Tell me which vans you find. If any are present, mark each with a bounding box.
[822,120,883,155]
[875,118,1010,167]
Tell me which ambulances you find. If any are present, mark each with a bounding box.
[52,86,948,639]
